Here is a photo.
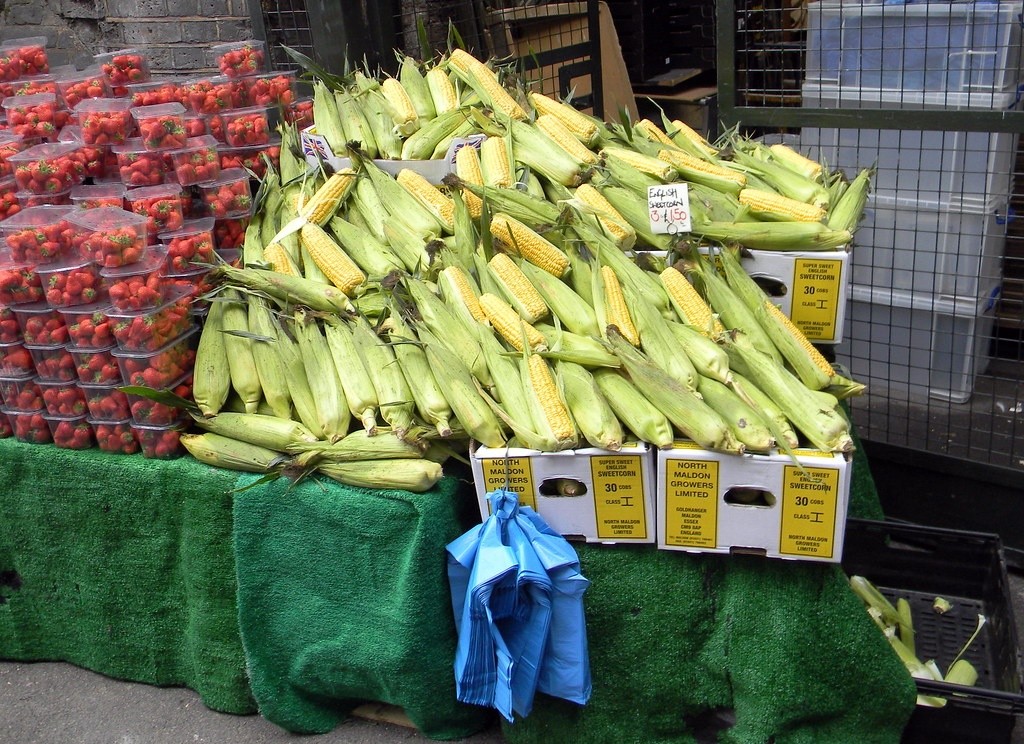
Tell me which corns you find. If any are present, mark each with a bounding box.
[179,49,875,493]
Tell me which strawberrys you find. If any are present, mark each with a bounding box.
[0,44,314,461]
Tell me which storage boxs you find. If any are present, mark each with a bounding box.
[2,2,1021,744]
